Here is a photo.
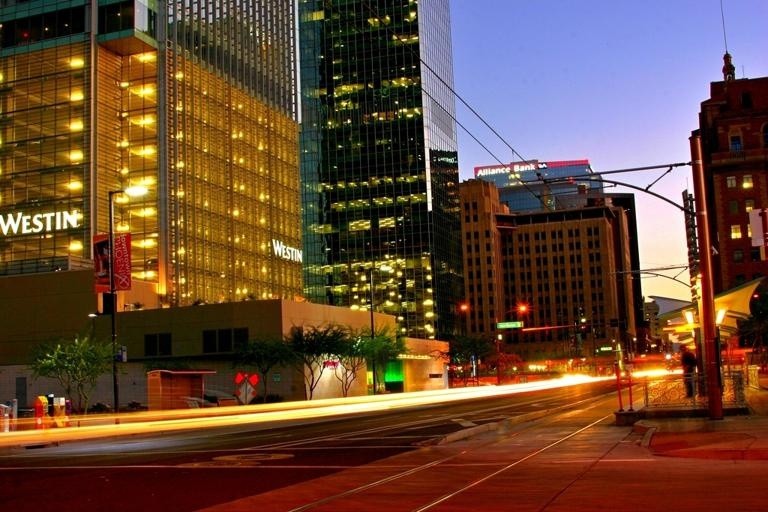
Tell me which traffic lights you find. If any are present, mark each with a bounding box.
[578,310,586,330]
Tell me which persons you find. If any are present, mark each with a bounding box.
[679,344,697,398]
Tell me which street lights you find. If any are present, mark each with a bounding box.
[105,186,154,412]
[368,265,392,396]
[497,302,527,380]
